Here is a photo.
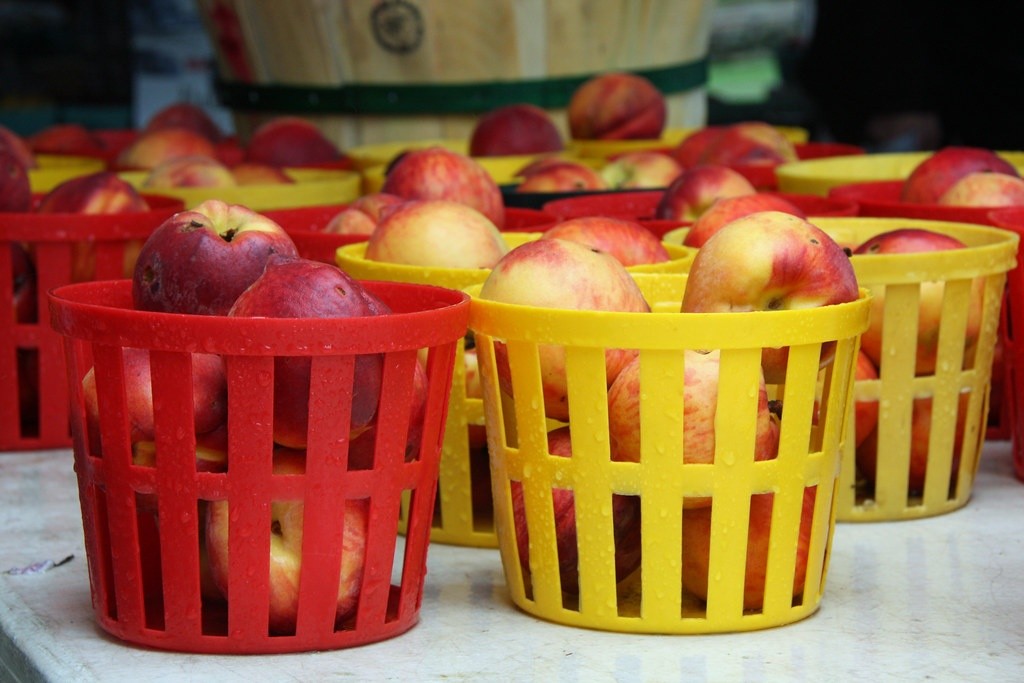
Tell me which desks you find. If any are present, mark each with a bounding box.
[0,441,1024,683]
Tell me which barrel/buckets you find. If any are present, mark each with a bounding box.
[195,0,708,152]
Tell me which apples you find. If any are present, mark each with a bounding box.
[0,73,1024,630]
